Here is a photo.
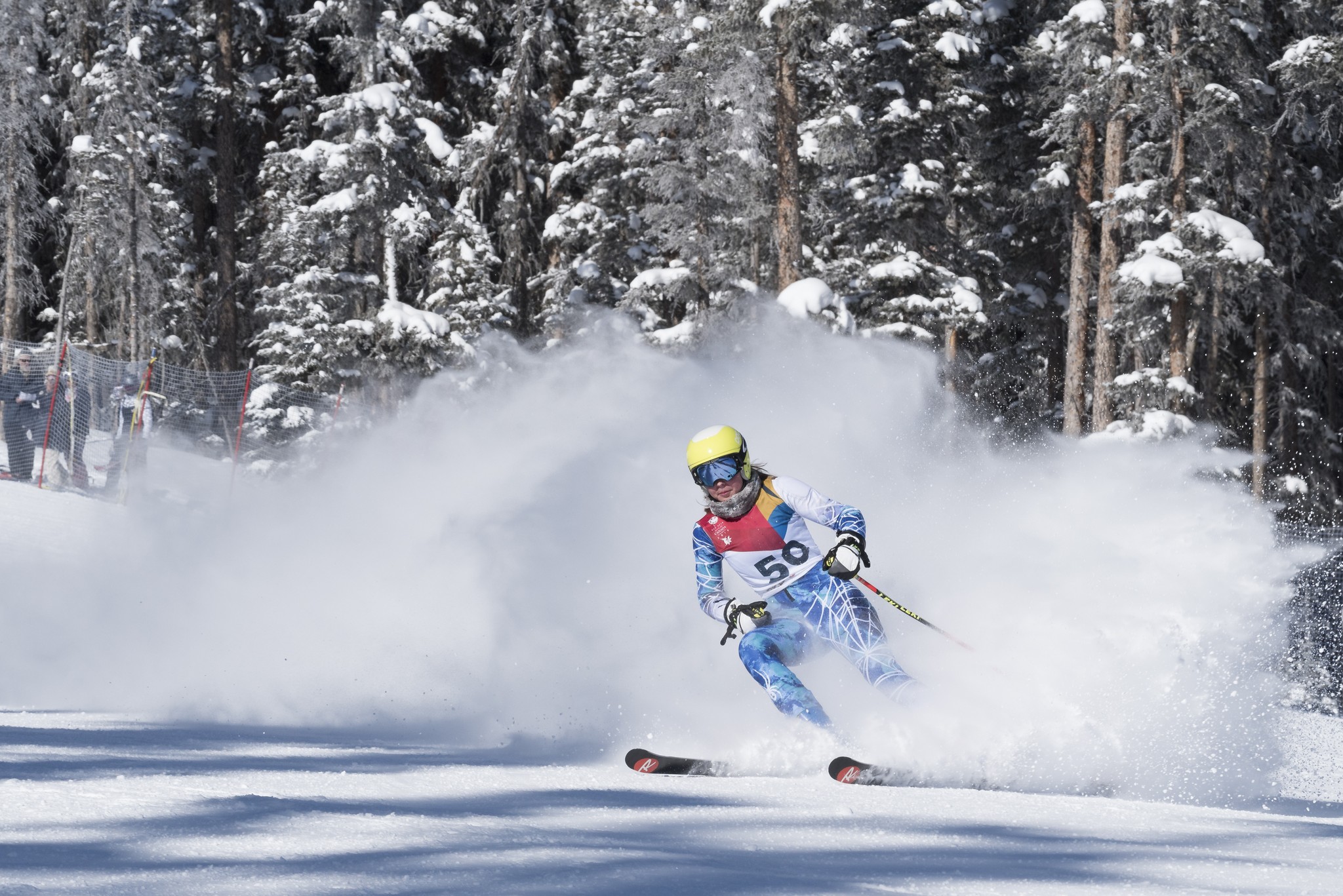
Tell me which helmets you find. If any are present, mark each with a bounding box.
[688,426,751,481]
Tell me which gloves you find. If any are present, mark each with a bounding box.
[822,538,862,580]
[734,601,772,636]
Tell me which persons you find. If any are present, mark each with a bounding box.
[686,424,916,758]
[0,348,91,487]
[99,374,152,493]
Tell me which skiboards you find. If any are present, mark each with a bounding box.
[624,747,1009,790]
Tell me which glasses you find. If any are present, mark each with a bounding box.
[697,457,741,486]
